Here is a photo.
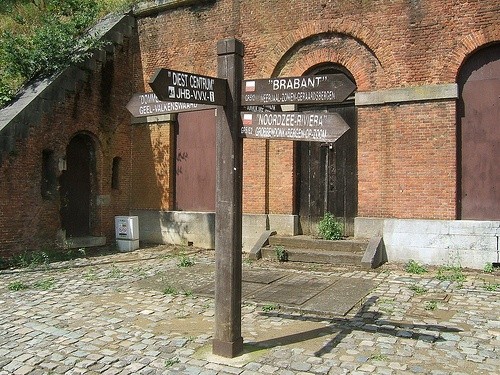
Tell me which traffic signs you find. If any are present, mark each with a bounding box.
[242,103,284,113]
[239,111,351,142]
[125,92,218,119]
[147,68,227,106]
[242,73,358,106]
